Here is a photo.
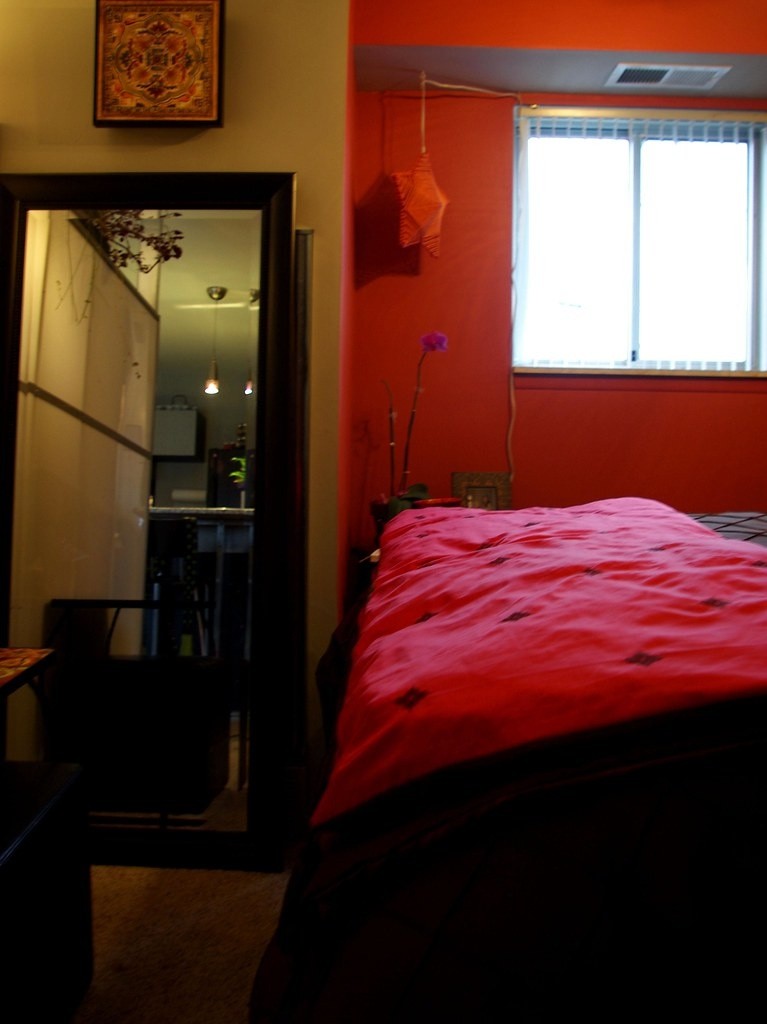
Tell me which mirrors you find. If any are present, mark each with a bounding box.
[0,171,309,870]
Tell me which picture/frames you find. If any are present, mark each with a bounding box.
[451,469,514,512]
[93,0,225,129]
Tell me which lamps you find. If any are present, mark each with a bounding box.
[205,286,228,394]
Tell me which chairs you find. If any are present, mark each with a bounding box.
[0,647,59,820]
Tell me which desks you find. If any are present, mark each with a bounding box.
[50,596,220,835]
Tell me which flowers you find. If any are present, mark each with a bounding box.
[81,206,185,273]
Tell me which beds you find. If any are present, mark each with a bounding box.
[247,497,767,1024]
